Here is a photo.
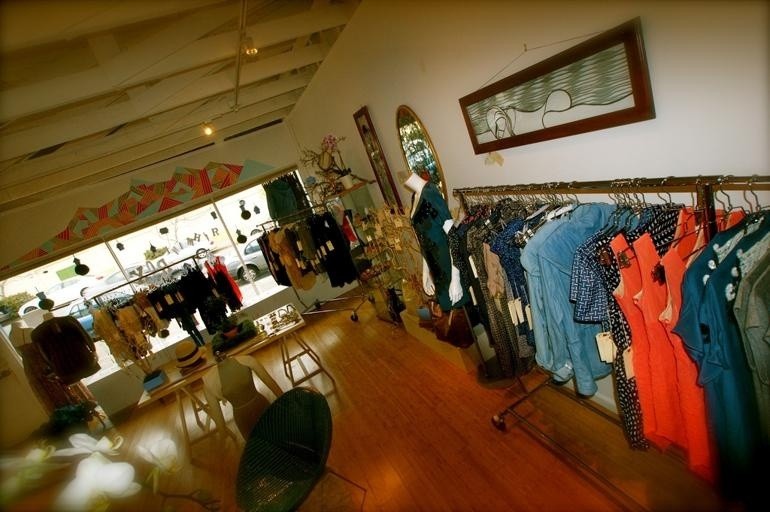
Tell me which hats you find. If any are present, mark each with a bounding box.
[175,341,207,367]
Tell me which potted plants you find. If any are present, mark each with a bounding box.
[0,292,35,319]
[332,168,353,190]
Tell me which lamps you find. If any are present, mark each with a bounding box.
[244,36,258,56]
[203,122,215,136]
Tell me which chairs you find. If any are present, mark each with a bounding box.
[235,387,367,512]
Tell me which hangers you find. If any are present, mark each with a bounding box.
[257,202,327,244]
[98,248,226,313]
[452,175,761,286]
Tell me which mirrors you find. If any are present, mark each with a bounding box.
[398,109,444,199]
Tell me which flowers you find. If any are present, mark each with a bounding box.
[320,134,347,168]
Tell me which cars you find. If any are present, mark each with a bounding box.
[89,263,200,306]
[225,230,280,283]
[63,283,150,341]
[18,273,108,320]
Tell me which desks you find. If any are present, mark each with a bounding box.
[138,302,335,458]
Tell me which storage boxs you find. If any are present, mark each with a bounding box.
[473,323,496,362]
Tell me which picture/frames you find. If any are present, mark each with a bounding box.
[352,105,405,215]
[458,16,656,155]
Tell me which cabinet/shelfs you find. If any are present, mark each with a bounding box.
[137,305,335,407]
[321,181,375,244]
